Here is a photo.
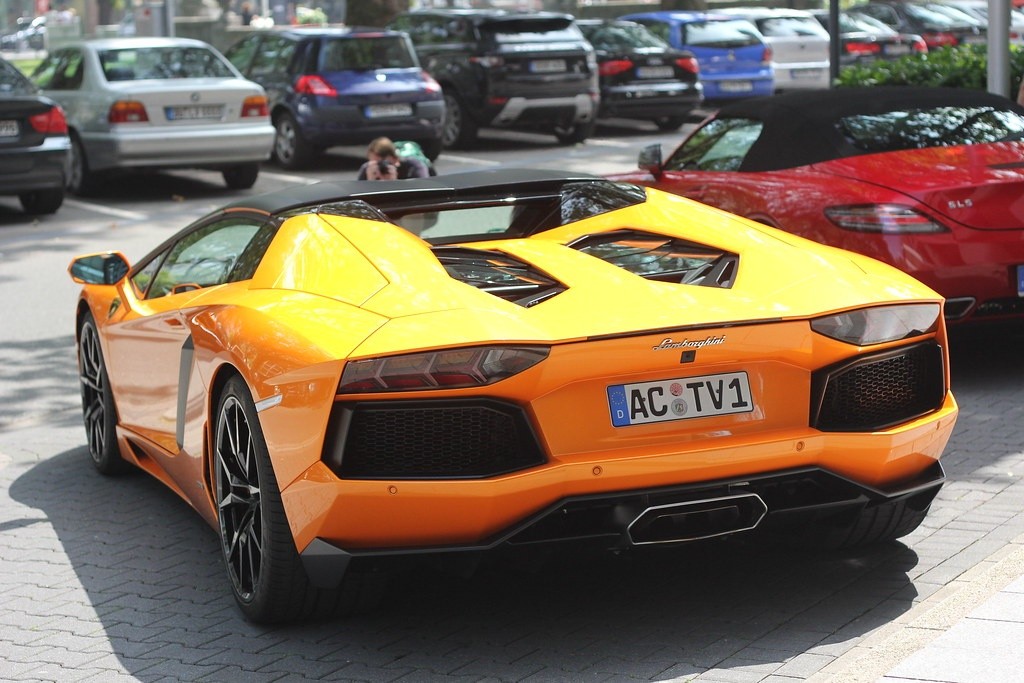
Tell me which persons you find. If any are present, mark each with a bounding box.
[366,136,439,238]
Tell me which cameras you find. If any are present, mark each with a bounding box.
[378,160,392,173]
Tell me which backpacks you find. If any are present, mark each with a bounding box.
[393,141,431,171]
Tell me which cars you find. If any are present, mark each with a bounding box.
[67,168,959,625]
[374,8,598,147]
[805,1,1024,75]
[576,19,704,133]
[0,57,72,214]
[597,86,1023,333]
[212,29,445,161]
[721,2,831,90]
[33,36,275,189]
[623,11,775,121]
